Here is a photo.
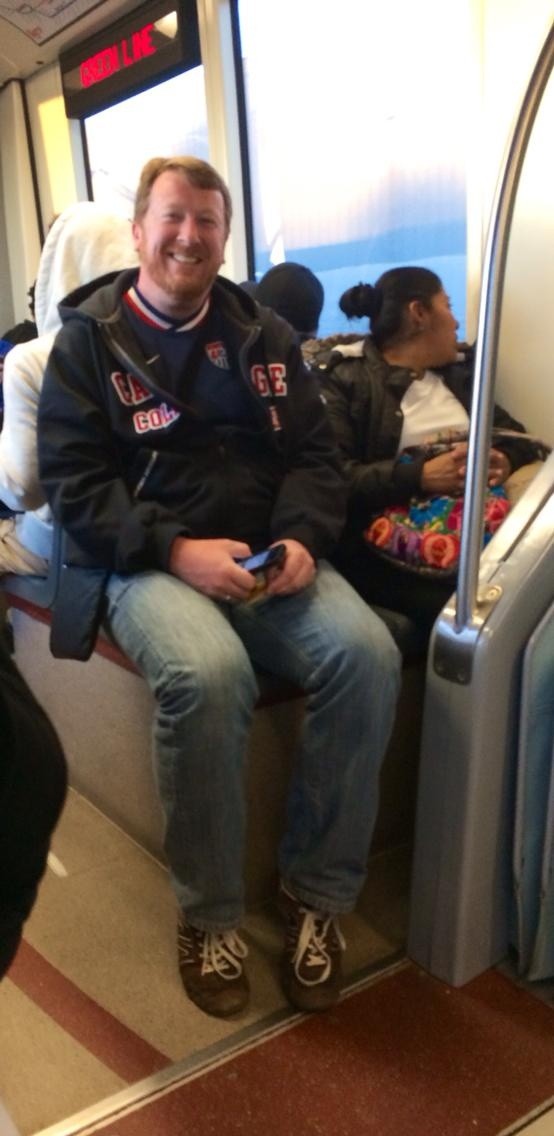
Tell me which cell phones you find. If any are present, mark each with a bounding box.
[236,543,287,573]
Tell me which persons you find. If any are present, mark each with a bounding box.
[0,205,139,584]
[297,268,545,630]
[236,262,326,340]
[39,156,404,1017]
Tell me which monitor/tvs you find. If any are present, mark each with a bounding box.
[57,0,201,120]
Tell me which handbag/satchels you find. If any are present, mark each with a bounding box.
[360,442,512,583]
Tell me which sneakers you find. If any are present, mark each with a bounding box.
[176,911,251,1017]
[277,879,345,1013]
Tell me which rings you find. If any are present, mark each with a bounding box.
[224,593,230,601]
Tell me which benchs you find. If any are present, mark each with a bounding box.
[1,569,431,916]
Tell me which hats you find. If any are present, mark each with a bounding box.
[236,262,324,332]
